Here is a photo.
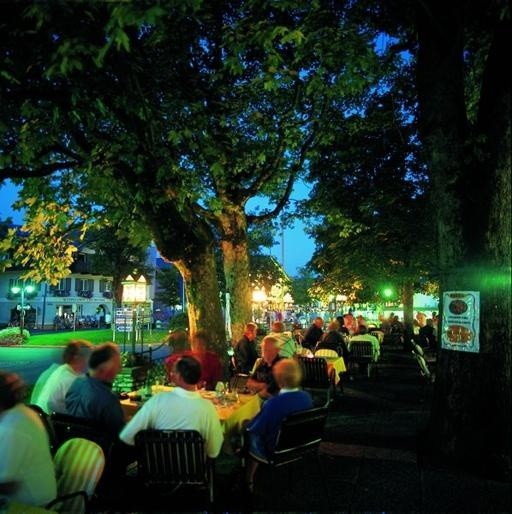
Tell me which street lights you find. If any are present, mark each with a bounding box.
[12,285,34,334]
[253,290,265,329]
[336,295,346,315]
[120,269,151,359]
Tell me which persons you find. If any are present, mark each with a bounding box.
[54,305,112,330]
[164,328,224,391]
[31,340,124,428]
[0,370,59,513]
[229,358,311,491]
[231,304,440,386]
[119,354,225,498]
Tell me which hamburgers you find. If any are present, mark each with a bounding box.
[447,325,472,343]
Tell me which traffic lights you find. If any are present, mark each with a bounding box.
[384,289,393,297]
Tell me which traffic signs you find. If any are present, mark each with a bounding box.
[112,308,140,333]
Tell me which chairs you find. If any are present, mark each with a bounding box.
[49,411,137,506]
[44,438,105,511]
[134,428,216,509]
[411,338,438,404]
[29,404,55,453]
[148,334,382,404]
[233,406,327,506]
